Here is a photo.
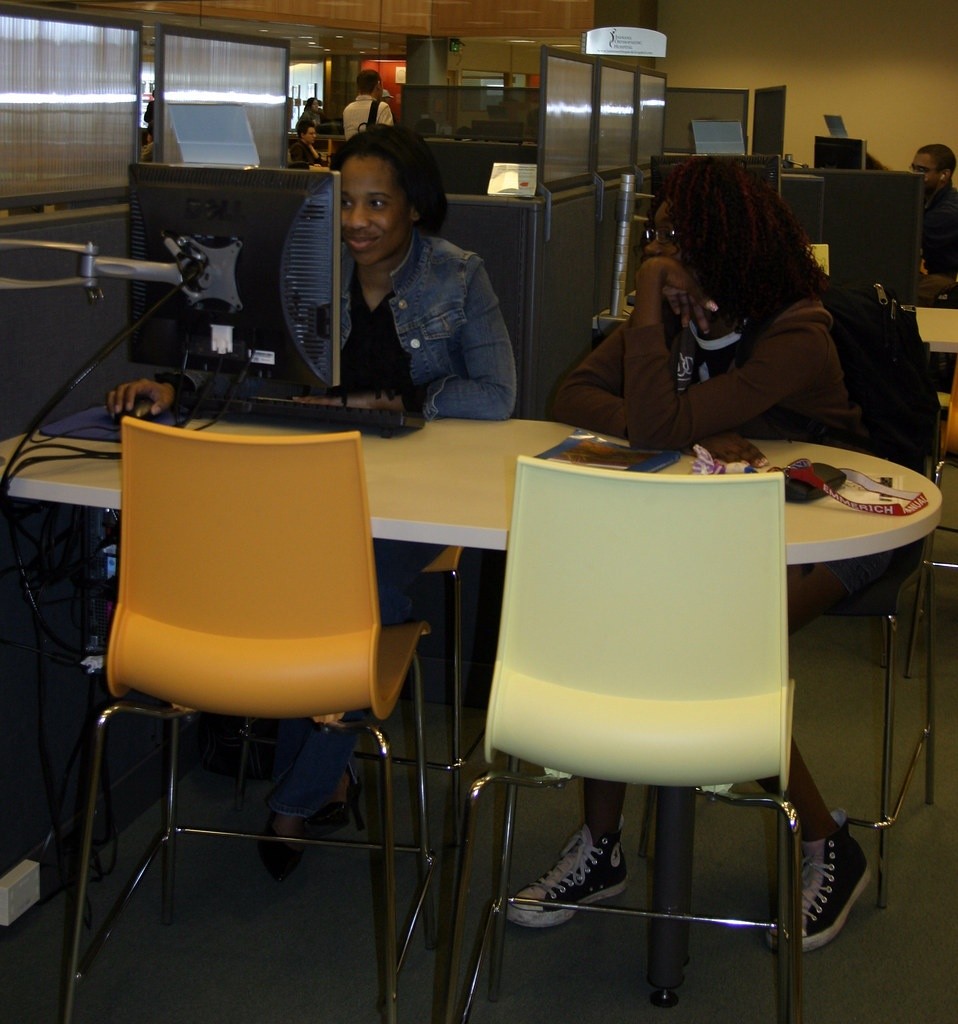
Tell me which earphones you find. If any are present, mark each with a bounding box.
[940,174,946,181]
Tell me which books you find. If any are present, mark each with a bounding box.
[534,428,680,471]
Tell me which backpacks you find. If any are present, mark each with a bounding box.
[821,280,941,475]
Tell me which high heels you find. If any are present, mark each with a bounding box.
[260,808,307,884]
[306,763,365,840]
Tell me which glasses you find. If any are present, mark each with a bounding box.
[645,227,680,246]
[909,164,943,174]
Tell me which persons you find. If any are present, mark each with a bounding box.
[140,90,155,160]
[289,120,328,167]
[909,144,958,306]
[106,123,517,884]
[343,69,394,141]
[506,153,872,957]
[295,97,327,130]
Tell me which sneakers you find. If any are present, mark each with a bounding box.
[507,811,629,928]
[766,808,871,952]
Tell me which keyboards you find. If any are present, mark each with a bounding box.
[171,390,427,438]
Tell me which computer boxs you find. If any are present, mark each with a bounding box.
[80,505,122,676]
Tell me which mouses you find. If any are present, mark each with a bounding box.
[113,397,159,425]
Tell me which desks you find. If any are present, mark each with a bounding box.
[289,133,347,168]
[597,291,958,455]
[0,409,943,1008]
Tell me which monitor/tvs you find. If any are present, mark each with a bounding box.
[124,161,340,386]
[651,153,780,243]
[815,136,868,171]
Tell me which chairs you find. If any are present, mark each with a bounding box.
[454,455,805,1024]
[59,411,440,1024]
[903,356,957,680]
